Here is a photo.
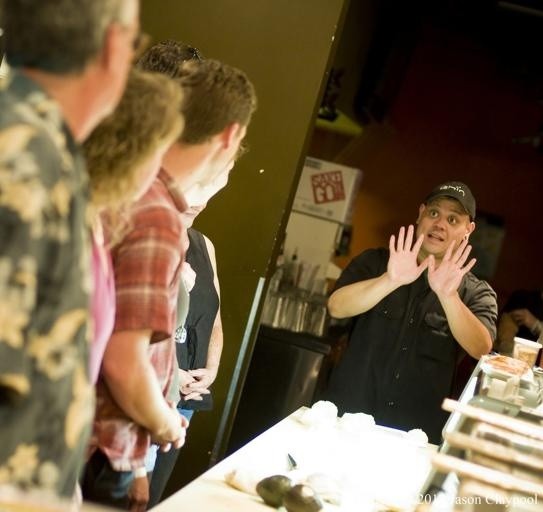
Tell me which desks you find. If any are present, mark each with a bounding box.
[146,405,441,512]
[225,323,352,459]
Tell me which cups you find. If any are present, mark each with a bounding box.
[513,337,543,370]
[259,291,327,340]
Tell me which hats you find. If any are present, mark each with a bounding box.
[425,181,479,220]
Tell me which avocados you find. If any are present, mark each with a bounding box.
[255,475,292,507]
[284,484,324,512]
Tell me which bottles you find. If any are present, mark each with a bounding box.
[267,229,333,300]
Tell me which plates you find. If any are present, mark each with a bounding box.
[479,354,535,384]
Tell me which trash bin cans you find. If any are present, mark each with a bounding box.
[224,322,331,458]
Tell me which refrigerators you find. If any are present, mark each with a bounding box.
[277,156,362,285]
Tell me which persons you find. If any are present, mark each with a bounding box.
[326,181,499,447]
[1,0,258,512]
[497,288,543,358]
